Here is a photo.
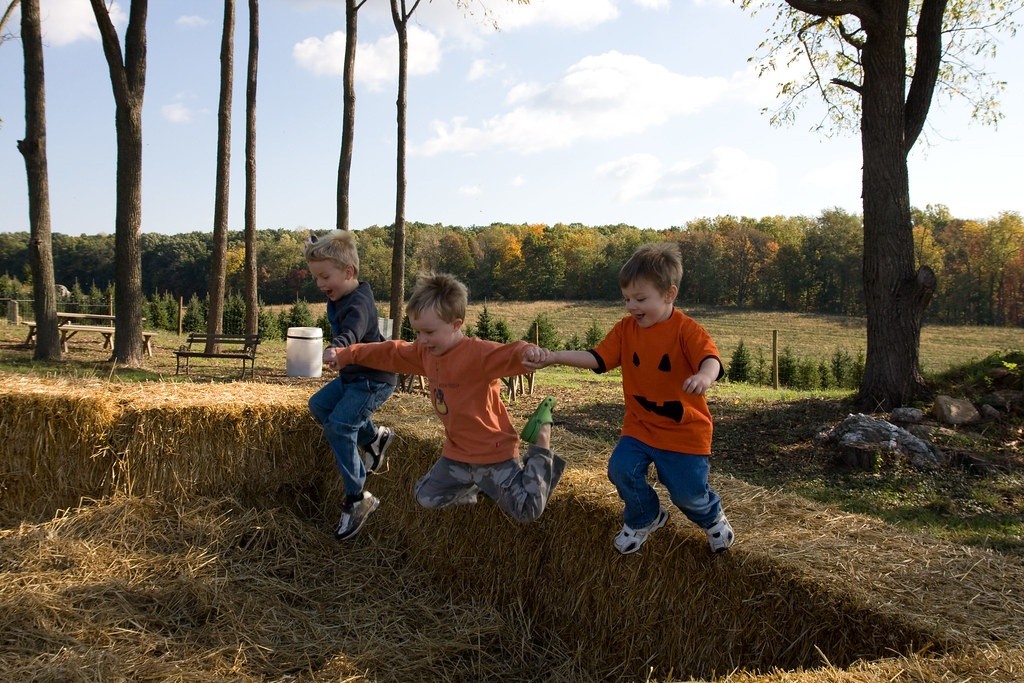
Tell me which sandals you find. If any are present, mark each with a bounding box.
[520,397,556,442]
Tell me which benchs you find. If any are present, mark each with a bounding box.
[21,321,158,356]
[172,332,262,379]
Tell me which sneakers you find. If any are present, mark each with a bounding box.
[334,490,380,540]
[613,505,668,554]
[705,508,735,553]
[363,426,394,478]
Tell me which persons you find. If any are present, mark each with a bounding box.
[321,275,567,523]
[522,245,735,556]
[301,229,398,542]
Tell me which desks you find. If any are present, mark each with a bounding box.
[57,312,146,345]
